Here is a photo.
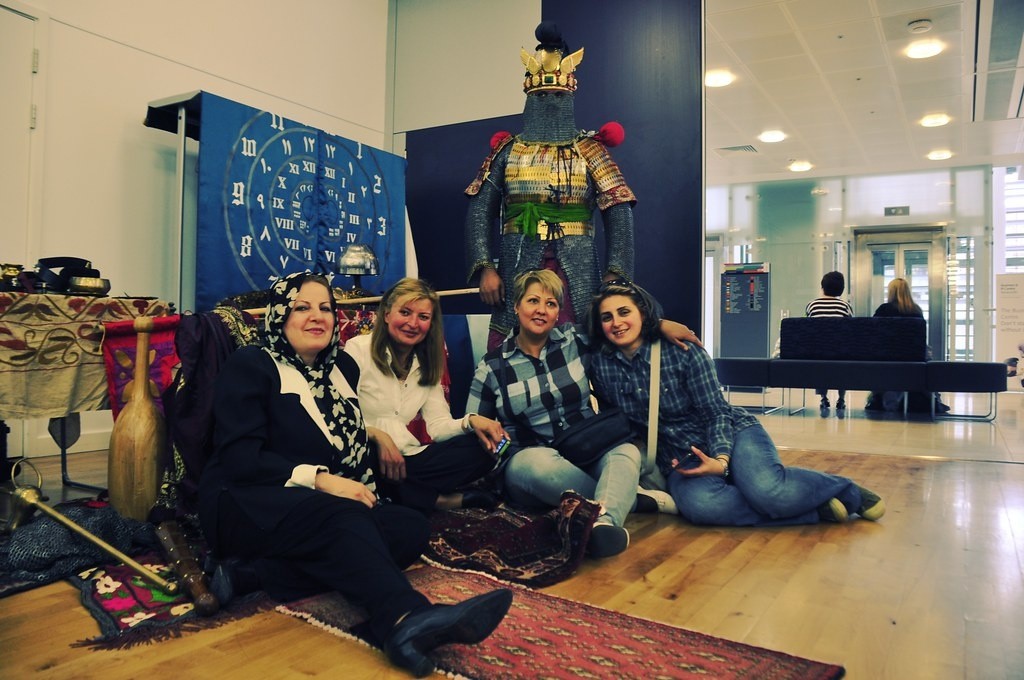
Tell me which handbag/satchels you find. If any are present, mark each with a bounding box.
[551,406,639,467]
[635,440,668,491]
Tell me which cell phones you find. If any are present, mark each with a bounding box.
[495,439,510,456]
[674,452,696,470]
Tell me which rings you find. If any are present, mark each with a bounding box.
[500,434,503,437]
[688,329,696,337]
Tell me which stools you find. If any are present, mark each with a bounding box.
[927,360,1008,423]
[713,357,784,414]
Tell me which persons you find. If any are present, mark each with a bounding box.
[873,279,924,318]
[805,271,853,409]
[342,278,511,510]
[467,18,636,356]
[201,272,513,680]
[465,267,678,557]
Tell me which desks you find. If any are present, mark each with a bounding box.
[0,292,169,492]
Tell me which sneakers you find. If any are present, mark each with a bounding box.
[816,498,849,524]
[850,482,887,521]
[636,485,679,515]
[588,515,631,558]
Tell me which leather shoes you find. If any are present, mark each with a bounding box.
[454,488,496,511]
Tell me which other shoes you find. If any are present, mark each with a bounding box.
[819,398,830,408]
[836,400,846,409]
[865,401,884,410]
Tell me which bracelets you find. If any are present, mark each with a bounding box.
[658,316,663,325]
[716,457,729,463]
[585,279,885,525]
[462,409,478,432]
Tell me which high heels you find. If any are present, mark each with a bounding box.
[386,588,513,679]
[203,552,246,609]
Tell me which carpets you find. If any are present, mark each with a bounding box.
[0,490,845,680]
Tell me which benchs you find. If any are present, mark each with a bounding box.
[767,317,927,418]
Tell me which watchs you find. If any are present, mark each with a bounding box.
[717,460,729,478]
